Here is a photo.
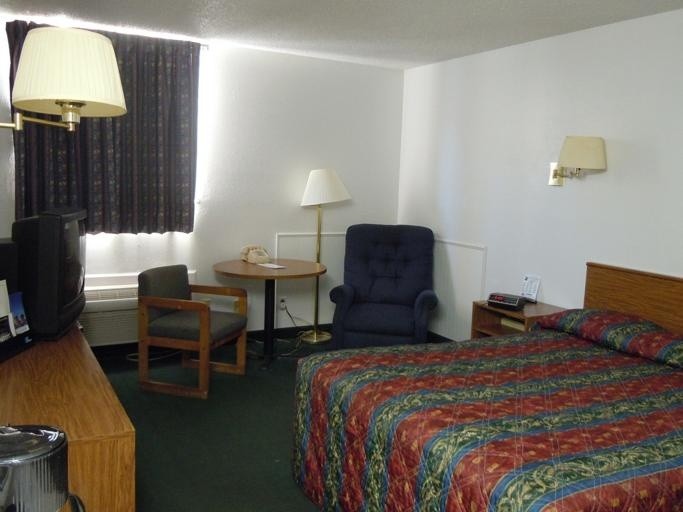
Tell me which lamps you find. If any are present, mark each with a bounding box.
[548,135,606,186]
[0,26,128,132]
[297,166,351,346]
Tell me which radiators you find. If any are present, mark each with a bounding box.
[79,271,196,346]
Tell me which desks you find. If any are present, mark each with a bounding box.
[0,321,137,511]
[212,256,327,372]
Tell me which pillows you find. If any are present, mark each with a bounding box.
[531,305,682,369]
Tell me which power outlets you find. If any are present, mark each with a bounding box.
[280,296,288,309]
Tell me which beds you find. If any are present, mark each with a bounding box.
[292,261,682,512]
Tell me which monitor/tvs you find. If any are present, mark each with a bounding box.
[0,207,86,342]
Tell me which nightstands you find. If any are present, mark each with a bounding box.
[470,299,569,341]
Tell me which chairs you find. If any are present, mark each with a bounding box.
[138,265,247,399]
[328,222,440,351]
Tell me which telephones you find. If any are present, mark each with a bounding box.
[241,244,272,264]
